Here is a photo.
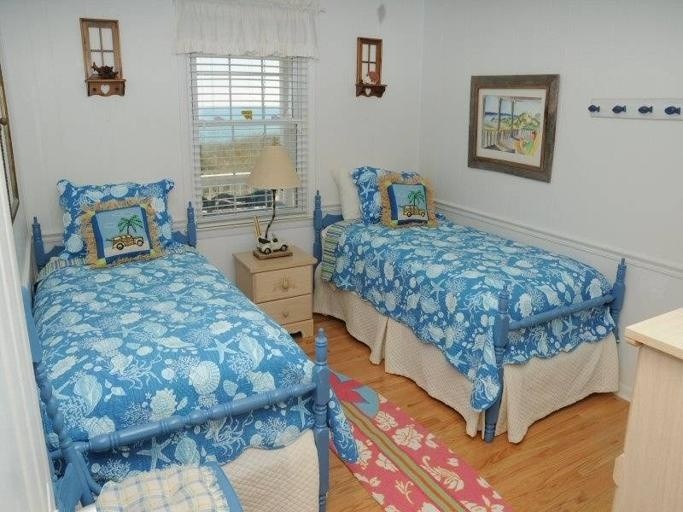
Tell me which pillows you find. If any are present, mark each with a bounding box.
[330,164,441,229]
[56,178,174,269]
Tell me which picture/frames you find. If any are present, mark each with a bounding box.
[466,72,561,183]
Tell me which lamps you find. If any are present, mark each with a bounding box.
[247,144,304,260]
